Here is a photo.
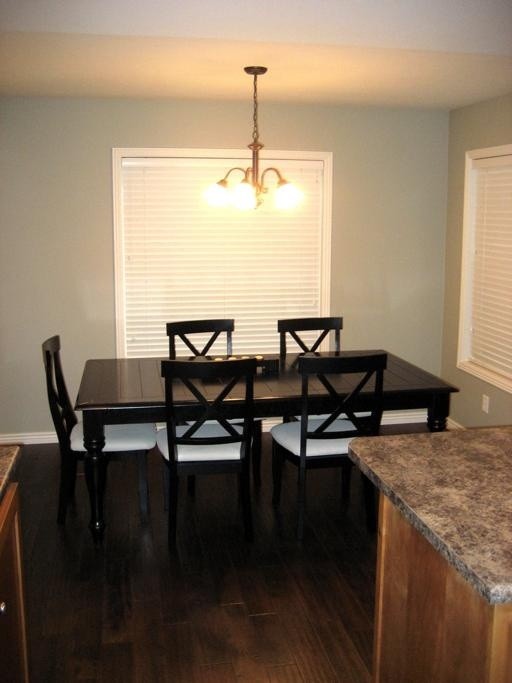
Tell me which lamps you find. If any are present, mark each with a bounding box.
[203,64,307,216]
